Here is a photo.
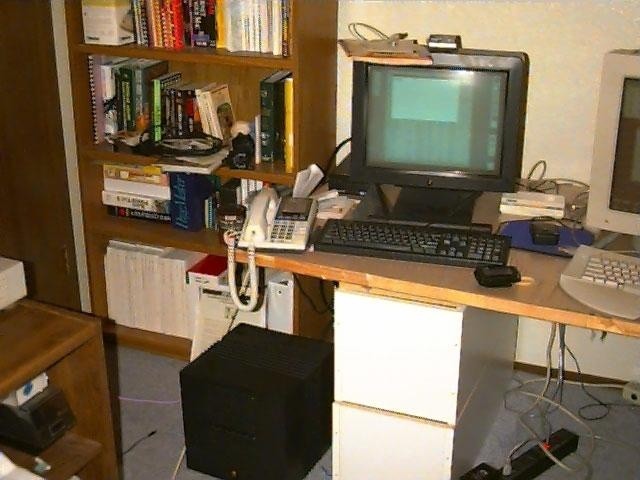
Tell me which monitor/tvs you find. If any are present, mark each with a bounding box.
[586,48,640,237]
[351,47,530,234]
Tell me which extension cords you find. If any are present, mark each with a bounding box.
[496,427,579,480]
[623,382,640,406]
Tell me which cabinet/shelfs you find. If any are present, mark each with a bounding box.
[62,0,339,366]
[1,299,120,479]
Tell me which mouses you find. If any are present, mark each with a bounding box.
[530,219,561,246]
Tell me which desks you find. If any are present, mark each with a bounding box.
[230,177,640,479]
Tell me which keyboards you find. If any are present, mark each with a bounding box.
[314,217,513,269]
[559,243,640,320]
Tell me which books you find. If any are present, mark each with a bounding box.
[104,238,268,364]
[294,164,353,220]
[499,191,566,220]
[429,36,461,49]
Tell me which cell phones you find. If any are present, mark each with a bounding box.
[474,266,521,288]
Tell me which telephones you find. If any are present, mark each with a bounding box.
[238,186,320,253]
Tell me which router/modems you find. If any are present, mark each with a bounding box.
[499,189,566,220]
